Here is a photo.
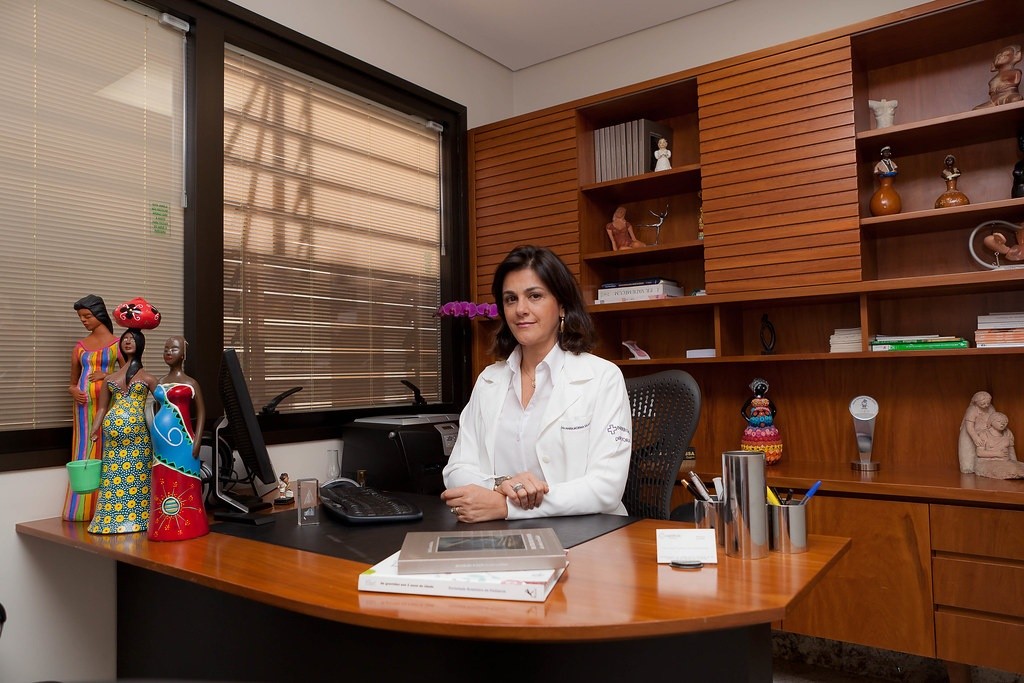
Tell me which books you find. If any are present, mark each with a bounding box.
[358,528,569,603]
[686,349,716,358]
[595,120,672,183]
[830,311,1024,353]
[594,278,684,304]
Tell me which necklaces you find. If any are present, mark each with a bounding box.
[522,368,535,388]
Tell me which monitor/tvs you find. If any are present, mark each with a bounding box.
[341,413,460,497]
[212,349,279,525]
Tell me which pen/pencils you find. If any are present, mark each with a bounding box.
[772,486,785,506]
[689,471,713,502]
[799,480,821,506]
[784,489,794,506]
[681,479,702,500]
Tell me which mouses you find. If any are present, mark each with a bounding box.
[319,477,361,489]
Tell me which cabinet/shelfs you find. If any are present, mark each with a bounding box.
[467,0,1024,675]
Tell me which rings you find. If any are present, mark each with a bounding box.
[456,507,461,515]
[512,482,524,492]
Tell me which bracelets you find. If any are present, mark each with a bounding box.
[495,476,511,490]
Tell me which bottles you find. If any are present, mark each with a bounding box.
[326,450,340,481]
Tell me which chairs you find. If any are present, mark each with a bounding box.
[621,369,702,520]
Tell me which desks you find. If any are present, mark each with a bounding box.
[16,481,852,683]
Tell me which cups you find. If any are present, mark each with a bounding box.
[695,495,724,547]
[769,499,807,554]
[65,459,102,494]
[721,449,769,559]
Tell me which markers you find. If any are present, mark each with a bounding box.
[767,486,780,506]
[712,477,724,502]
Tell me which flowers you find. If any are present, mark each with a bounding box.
[433,301,499,322]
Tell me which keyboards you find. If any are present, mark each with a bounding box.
[319,486,424,525]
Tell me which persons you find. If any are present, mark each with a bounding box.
[740,378,783,464]
[869,99,897,128]
[654,139,672,172]
[278,473,290,499]
[606,203,670,251]
[440,245,633,524]
[957,391,1024,479]
[873,146,898,177]
[973,44,1022,109]
[68,295,210,541]
[941,155,961,181]
